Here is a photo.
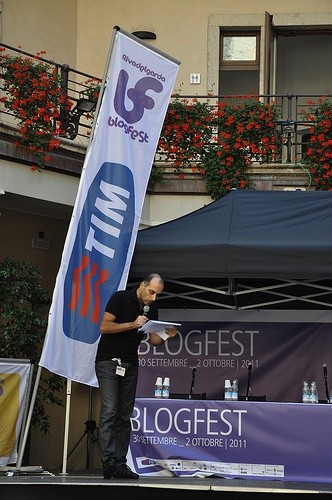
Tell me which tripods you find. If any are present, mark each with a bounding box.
[56,386,102,472]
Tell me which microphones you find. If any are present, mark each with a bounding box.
[186,368,197,400]
[140,304,150,328]
[244,364,252,401]
[323,363,331,403]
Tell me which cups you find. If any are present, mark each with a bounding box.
[155,377,162,386]
[163,377,170,386]
[225,380,232,388]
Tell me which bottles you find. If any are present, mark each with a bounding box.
[162,386,170,398]
[155,385,162,398]
[303,382,310,403]
[310,382,318,404]
[232,380,238,401]
[224,387,232,401]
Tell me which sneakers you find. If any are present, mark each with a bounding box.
[114,465,139,479]
[102,457,128,480]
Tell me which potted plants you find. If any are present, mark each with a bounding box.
[0,255,66,466]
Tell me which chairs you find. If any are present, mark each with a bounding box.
[300,400,328,404]
[237,394,266,402]
[167,392,206,401]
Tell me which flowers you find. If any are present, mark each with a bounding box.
[0,46,74,173]
[79,78,283,203]
[294,95,332,192]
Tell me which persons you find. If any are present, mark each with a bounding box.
[94,274,177,479]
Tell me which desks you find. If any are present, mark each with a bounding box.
[125,397,332,483]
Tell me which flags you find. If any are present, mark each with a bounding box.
[0,364,31,466]
[38,30,181,389]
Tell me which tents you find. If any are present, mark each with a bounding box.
[125,189,332,311]
